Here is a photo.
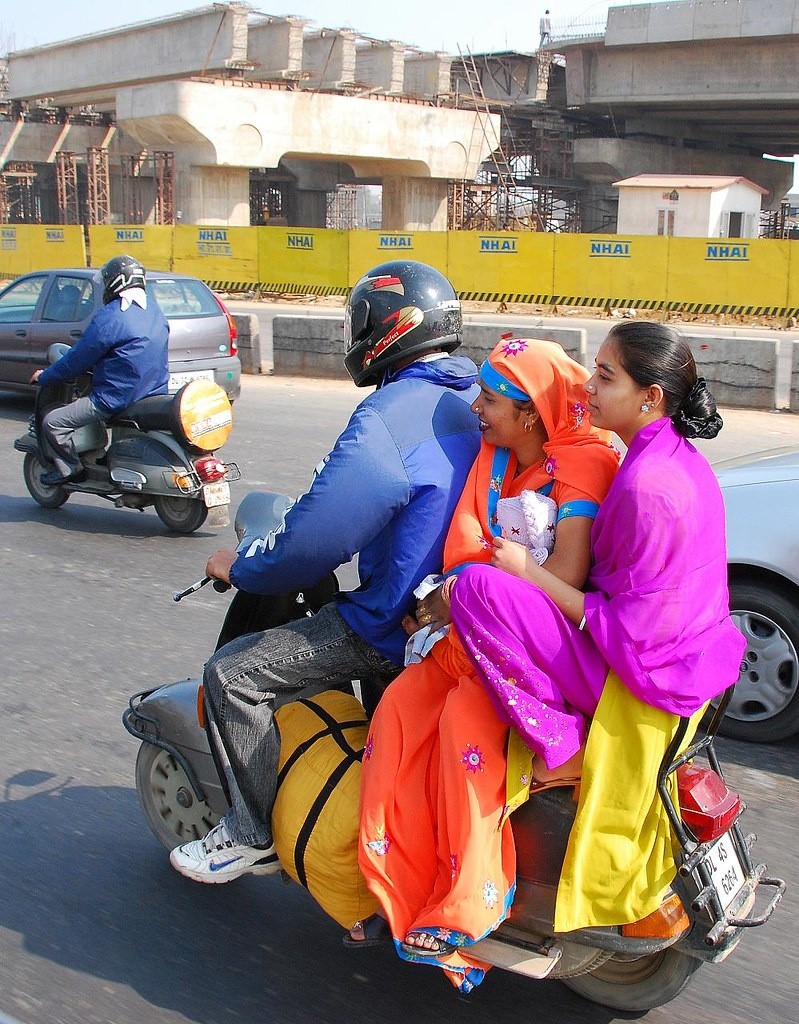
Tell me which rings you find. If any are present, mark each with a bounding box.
[422,614,431,624]
[420,605,427,615]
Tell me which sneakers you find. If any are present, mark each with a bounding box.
[40,468,86,484]
[168,817,283,885]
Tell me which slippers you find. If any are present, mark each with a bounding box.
[529,776,582,794]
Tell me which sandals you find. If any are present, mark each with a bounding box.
[400,932,458,957]
[342,913,394,948]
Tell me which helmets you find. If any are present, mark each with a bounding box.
[101,255,146,305]
[343,261,464,387]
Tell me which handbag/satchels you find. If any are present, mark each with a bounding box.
[270,689,382,928]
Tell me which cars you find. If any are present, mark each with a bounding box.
[700,446,799,745]
[0,269,243,405]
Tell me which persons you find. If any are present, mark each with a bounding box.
[342,339,622,996]
[449,323,747,933]
[30,256,170,486]
[169,260,483,886]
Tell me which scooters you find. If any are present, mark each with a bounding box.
[118,482,788,1011]
[12,340,243,535]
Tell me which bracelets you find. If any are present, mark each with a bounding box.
[440,574,458,607]
[579,616,587,631]
